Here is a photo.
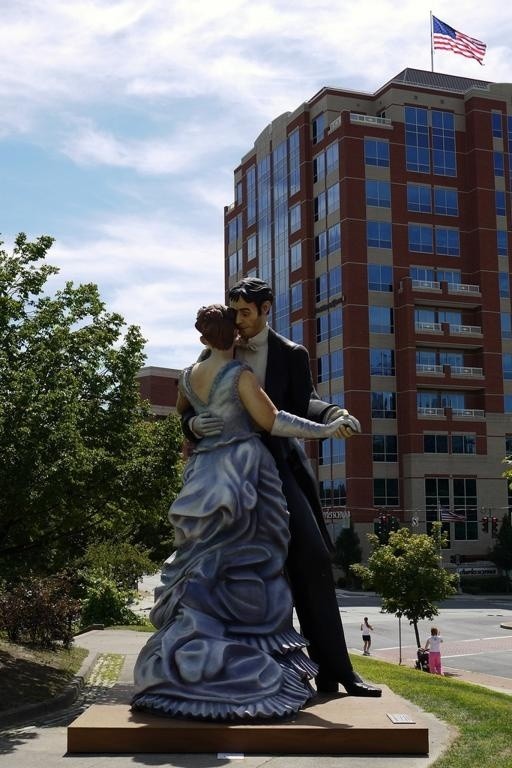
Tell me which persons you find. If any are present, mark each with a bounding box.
[181,276,382,698]
[360,617,374,655]
[420,627,444,675]
[175,302,362,700]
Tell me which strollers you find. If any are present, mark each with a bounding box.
[414,647,430,672]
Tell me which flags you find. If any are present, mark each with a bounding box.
[432,16,486,66]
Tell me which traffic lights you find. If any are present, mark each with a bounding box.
[491,517,498,539]
[374,515,388,545]
[389,515,400,533]
[481,516,489,534]
